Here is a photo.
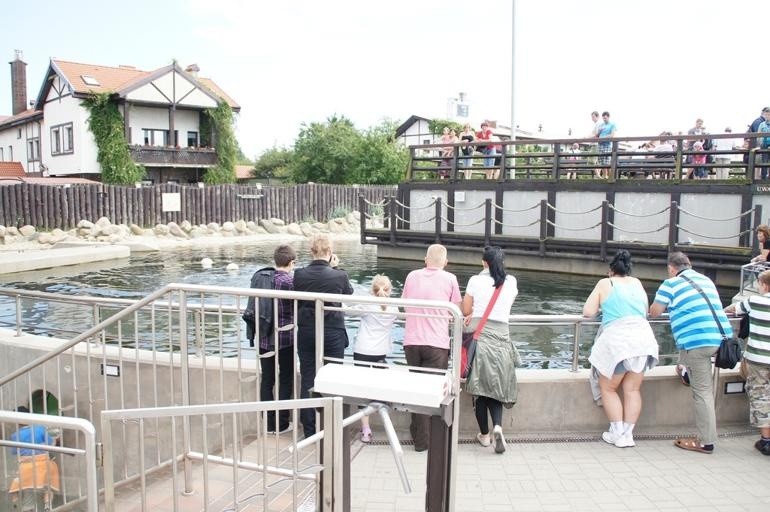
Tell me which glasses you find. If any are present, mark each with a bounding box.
[484,246,501,252]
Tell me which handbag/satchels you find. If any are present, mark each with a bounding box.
[461,334,478,378]
[716,338,742,369]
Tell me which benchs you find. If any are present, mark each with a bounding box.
[546,158,677,180]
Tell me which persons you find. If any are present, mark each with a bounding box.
[723,269,770,456]
[439,124,502,179]
[9,406,60,511]
[400,244,461,450]
[633,121,734,180]
[649,252,734,454]
[744,108,770,180]
[342,275,400,442]
[583,251,659,449]
[244,246,298,435]
[750,225,770,263]
[294,237,350,439]
[463,246,517,453]
[566,112,615,182]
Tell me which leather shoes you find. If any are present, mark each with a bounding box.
[756,440,770,455]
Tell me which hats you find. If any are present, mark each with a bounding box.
[693,142,702,148]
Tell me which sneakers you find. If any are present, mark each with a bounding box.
[602,431,627,448]
[273,422,293,434]
[360,428,372,443]
[476,431,490,448]
[308,387,325,412]
[492,425,507,454]
[624,431,636,447]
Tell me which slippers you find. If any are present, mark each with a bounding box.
[676,364,691,386]
[675,438,713,454]
